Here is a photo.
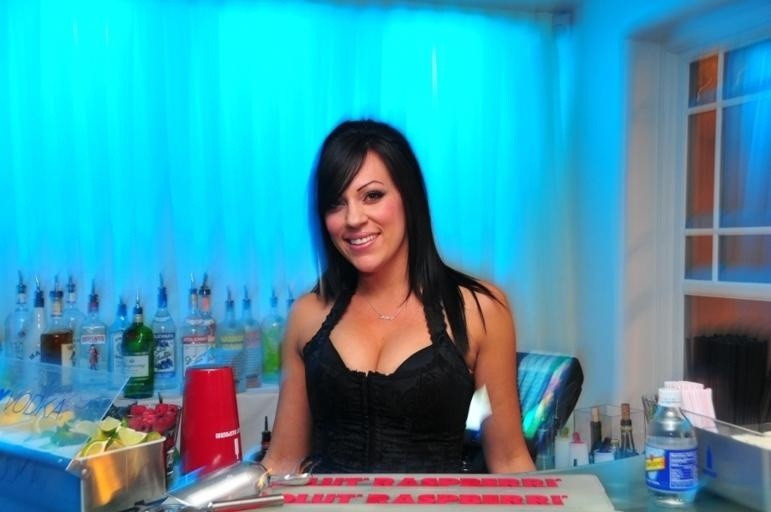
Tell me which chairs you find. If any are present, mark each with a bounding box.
[463,351,583,473]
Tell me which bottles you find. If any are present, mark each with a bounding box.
[6,268,300,396]
[643,388,699,512]
[234,438,240,460]
[261,416,272,457]
[534,402,637,470]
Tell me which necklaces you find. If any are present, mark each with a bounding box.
[356,280,413,321]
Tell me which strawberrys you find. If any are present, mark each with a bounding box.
[129,403,177,450]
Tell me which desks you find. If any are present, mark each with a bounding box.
[108,383,278,453]
[217,451,759,512]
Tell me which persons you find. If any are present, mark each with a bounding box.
[260,118,537,476]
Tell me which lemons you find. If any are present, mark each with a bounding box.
[81,416,161,458]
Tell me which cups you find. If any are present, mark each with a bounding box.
[181,367,241,476]
